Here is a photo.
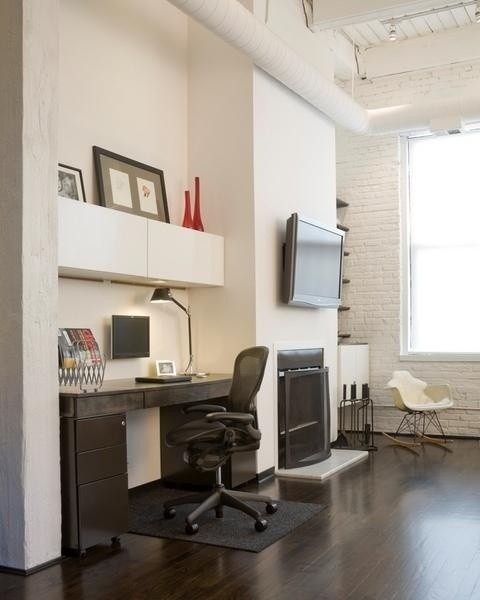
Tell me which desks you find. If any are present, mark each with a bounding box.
[60,371,259,561]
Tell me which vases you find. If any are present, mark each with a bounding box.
[182,190,193,229]
[192,176,205,233]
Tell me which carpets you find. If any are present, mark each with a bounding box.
[128,485,330,553]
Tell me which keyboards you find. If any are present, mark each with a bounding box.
[135,375,193,382]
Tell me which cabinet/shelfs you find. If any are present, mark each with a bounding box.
[60,413,131,562]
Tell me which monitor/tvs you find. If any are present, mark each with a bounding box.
[110,315,151,360]
[280,212,345,308]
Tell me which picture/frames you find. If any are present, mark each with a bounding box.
[92,145,170,224]
[58,162,87,203]
[155,359,177,377]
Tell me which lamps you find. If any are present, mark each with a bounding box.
[474,7,480,23]
[389,30,398,41]
[150,287,199,375]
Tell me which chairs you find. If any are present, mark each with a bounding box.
[162,345,278,535]
[381,369,455,448]
